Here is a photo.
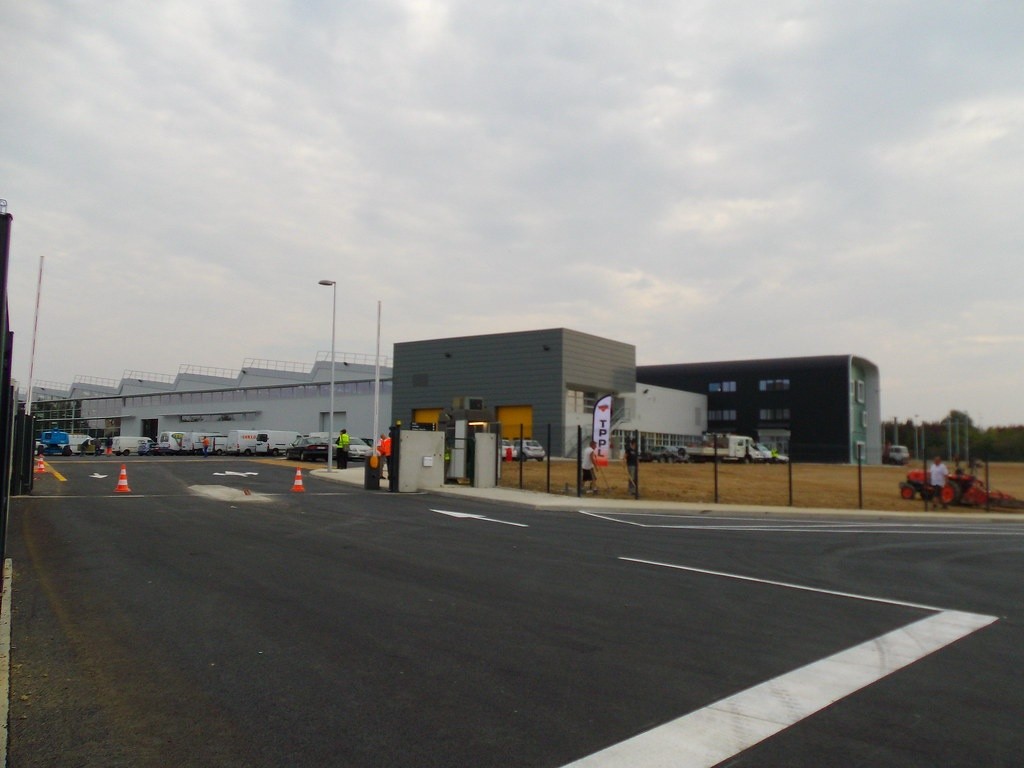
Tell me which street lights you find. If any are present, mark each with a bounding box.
[317,280,336,472]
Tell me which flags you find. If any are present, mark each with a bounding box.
[593,396,611,466]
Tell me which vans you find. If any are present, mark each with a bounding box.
[36,433,111,457]
[225,429,304,455]
[158,431,228,455]
[112,437,156,456]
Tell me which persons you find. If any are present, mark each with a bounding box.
[376,431,391,480]
[79,439,88,456]
[582,441,599,492]
[929,457,949,510]
[105,436,113,457]
[203,436,209,458]
[93,436,101,456]
[624,438,637,494]
[335,429,349,469]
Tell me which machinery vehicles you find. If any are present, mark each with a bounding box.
[898,461,1024,511]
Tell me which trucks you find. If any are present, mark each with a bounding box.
[887,445,909,464]
[684,434,753,463]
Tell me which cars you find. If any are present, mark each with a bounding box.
[749,442,789,463]
[501,439,546,461]
[285,435,374,462]
[646,446,689,463]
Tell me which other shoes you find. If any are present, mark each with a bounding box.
[586,490,594,494]
[592,486,597,491]
[382,476,386,479]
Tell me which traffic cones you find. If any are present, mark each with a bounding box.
[38,454,45,473]
[290,465,305,492]
[112,464,131,493]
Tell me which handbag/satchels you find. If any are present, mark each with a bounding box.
[343,444,350,452]
